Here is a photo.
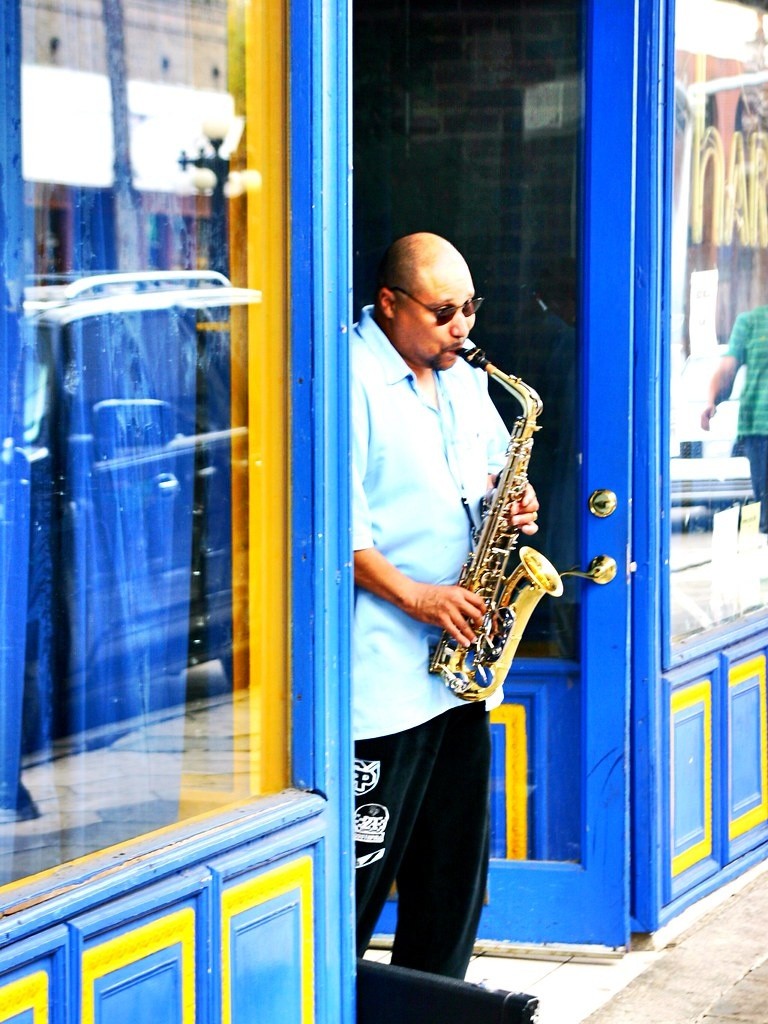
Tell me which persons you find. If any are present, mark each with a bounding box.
[701,305,768,584]
[351,231,542,981]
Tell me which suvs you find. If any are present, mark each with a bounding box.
[3,269,236,747]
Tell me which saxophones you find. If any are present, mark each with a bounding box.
[428,346,565,702]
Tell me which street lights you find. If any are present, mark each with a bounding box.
[171,119,238,270]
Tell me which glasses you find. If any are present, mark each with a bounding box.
[386,285,484,328]
